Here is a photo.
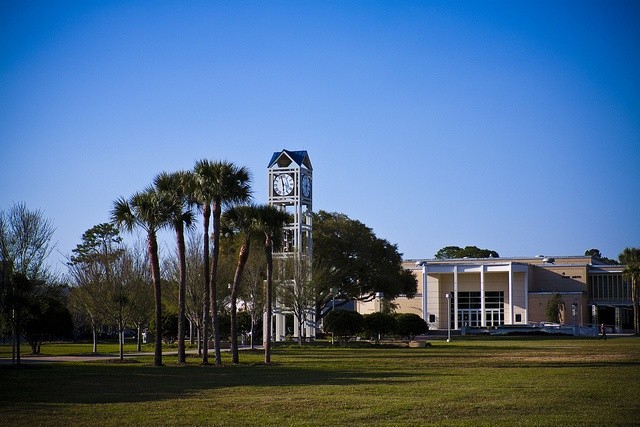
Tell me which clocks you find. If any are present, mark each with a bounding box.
[274,174,294,196]
[301,174,311,197]
